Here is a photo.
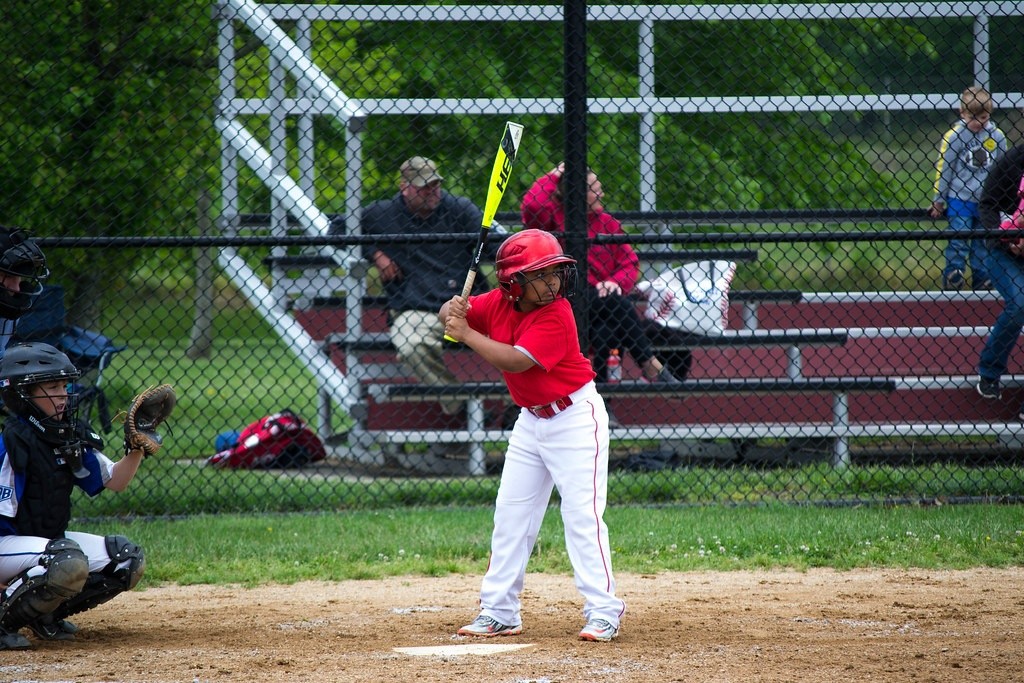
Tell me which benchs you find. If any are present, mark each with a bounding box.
[291,287,804,329]
[370,374,898,477]
[327,327,849,385]
[264,248,762,294]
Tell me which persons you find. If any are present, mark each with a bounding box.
[0,221,175,650]
[437,230,627,642]
[929,89,1010,289]
[973,144,1023,399]
[520,160,688,401]
[323,156,514,420]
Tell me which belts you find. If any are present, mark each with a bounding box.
[527,395,573,420]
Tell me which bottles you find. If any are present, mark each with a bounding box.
[607,349,621,383]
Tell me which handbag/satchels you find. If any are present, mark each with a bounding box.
[644,259,736,338]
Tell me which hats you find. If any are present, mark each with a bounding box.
[399,156,445,188]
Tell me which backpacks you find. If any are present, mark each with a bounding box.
[206,406,326,469]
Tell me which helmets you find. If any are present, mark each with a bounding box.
[495,228,578,306]
[0,227,50,321]
[0,341,80,445]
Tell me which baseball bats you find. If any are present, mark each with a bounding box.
[441,120,526,343]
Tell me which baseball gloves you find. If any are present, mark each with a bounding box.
[121,382,179,457]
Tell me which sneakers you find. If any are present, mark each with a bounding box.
[579,617,618,642]
[977,372,1002,400]
[457,614,523,638]
[0,624,33,651]
[25,616,78,641]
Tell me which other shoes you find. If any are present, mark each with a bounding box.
[945,270,966,291]
[977,279,993,291]
[639,368,681,385]
